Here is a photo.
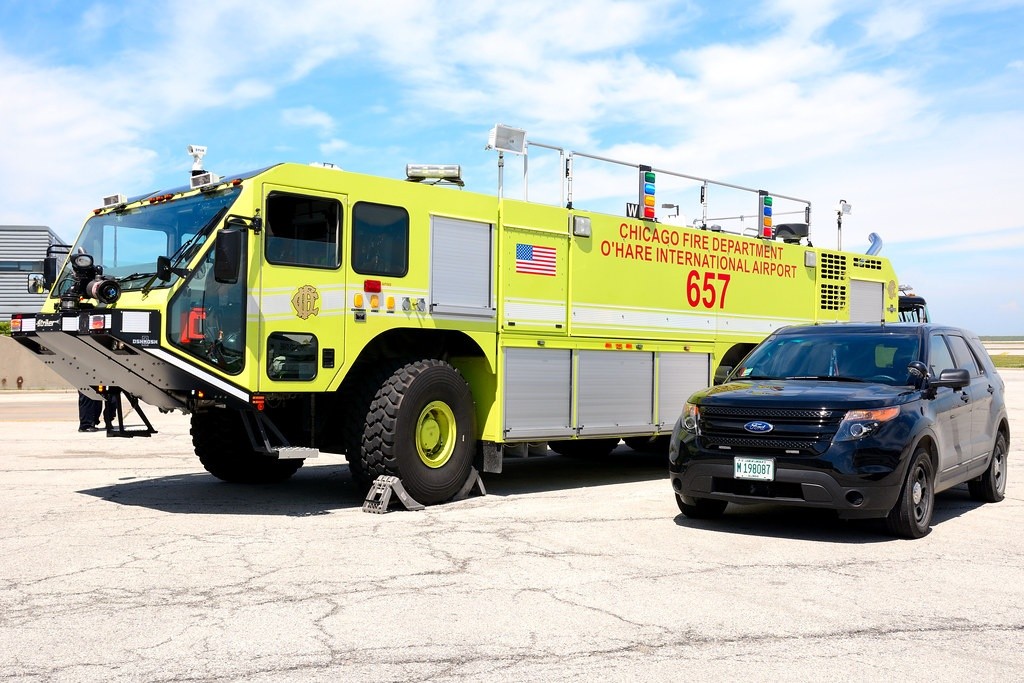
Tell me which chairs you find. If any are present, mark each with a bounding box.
[893,346,923,383]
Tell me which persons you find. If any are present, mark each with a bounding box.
[77,388,103,432]
[103,389,117,429]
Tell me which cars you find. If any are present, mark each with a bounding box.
[668,322,1010,540]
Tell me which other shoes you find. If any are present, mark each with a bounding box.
[78,427,99,432]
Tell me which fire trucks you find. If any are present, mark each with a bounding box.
[10,124,936,507]
